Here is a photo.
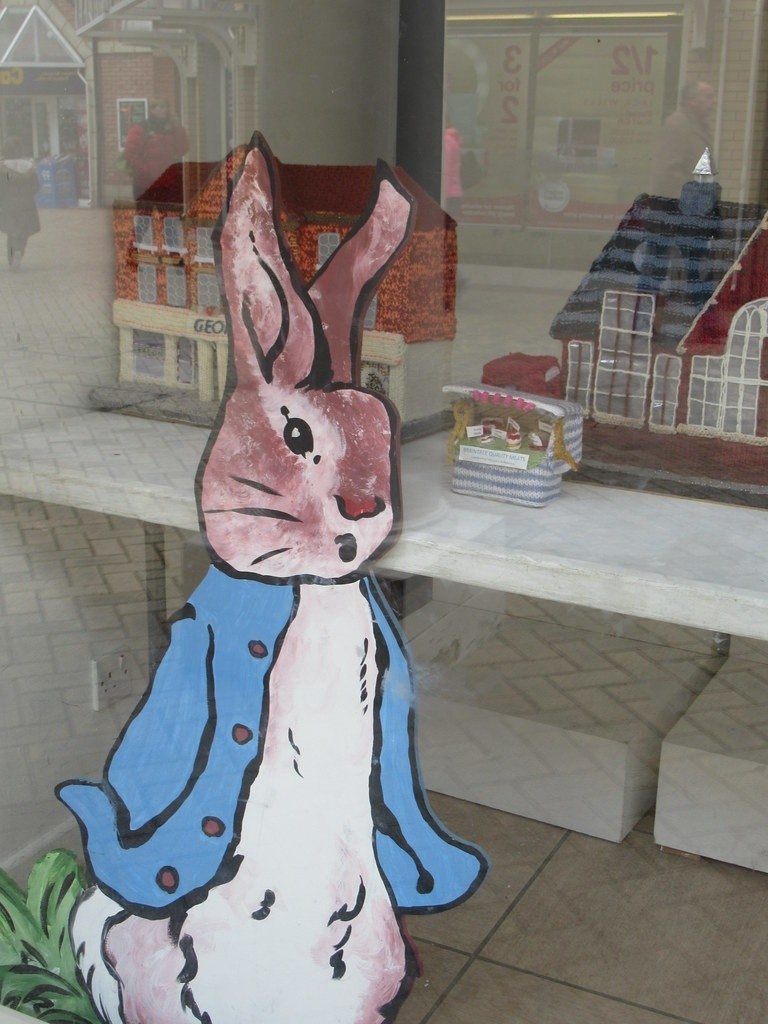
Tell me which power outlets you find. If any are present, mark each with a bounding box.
[90,647,133,712]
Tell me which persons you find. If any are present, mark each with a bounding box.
[630,81,731,344]
[0,134,41,269]
[127,96,190,277]
[443,113,464,215]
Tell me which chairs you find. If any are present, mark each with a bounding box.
[394,577,727,843]
[652,633,768,874]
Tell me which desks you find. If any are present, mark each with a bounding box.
[0,376,768,692]
